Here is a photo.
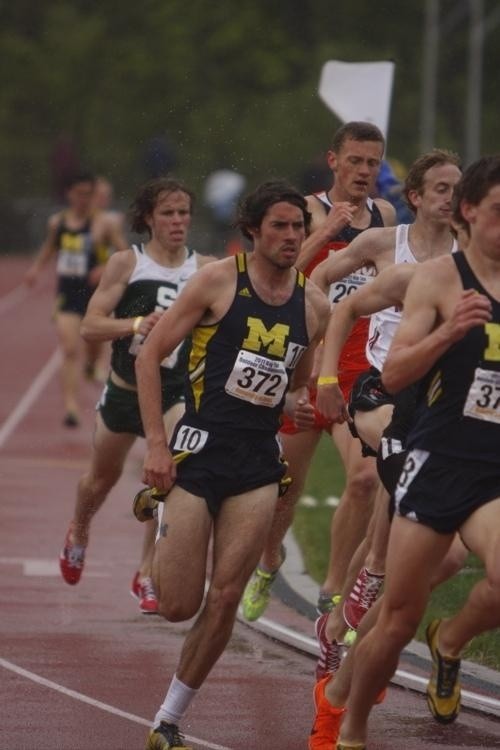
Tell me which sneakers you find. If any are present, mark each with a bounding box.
[59,519,89,586]
[426,617,462,725]
[342,565,386,632]
[314,613,346,683]
[132,485,160,522]
[373,688,387,704]
[315,591,357,647]
[308,676,348,750]
[242,543,287,622]
[62,402,79,428]
[336,735,366,750]
[81,358,111,384]
[129,570,159,613]
[145,720,194,750]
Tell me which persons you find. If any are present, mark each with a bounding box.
[340,158,500,750]
[240,119,398,646]
[90,177,127,379]
[132,179,332,750]
[26,165,125,427]
[376,158,422,222]
[58,181,222,615]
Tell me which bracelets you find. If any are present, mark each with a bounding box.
[315,375,336,383]
[135,312,143,330]
